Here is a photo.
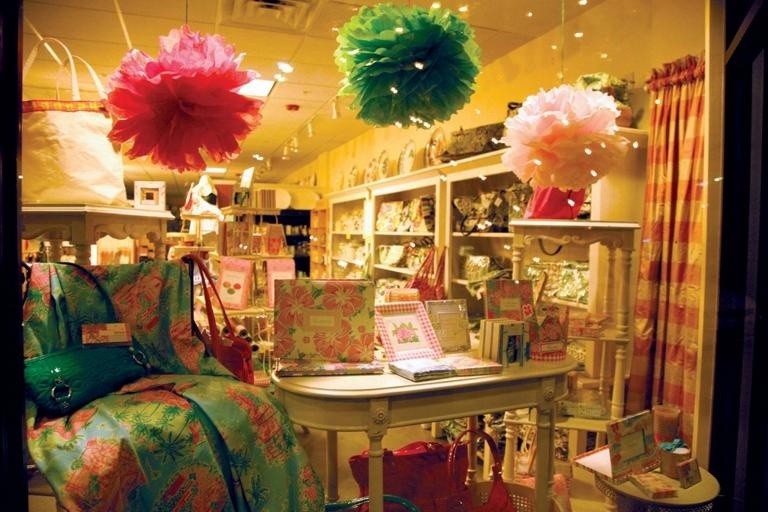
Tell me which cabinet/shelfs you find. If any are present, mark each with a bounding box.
[501,216,640,486]
[22,203,177,264]
[175,200,329,385]
[324,130,682,354]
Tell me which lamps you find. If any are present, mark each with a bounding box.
[263,101,338,172]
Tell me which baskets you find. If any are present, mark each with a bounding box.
[467,482,562,512]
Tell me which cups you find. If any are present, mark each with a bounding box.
[652,406,678,445]
[661,445,690,481]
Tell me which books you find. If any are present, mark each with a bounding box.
[433,353,503,376]
[253,189,277,209]
[490,321,525,370]
[476,318,510,359]
[482,320,518,364]
[388,356,456,382]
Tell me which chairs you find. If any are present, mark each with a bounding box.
[21,255,419,511]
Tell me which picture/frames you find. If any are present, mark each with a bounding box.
[345,126,448,187]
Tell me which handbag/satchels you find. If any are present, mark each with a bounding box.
[450,184,530,234]
[206,336,254,385]
[24,335,153,420]
[515,453,572,512]
[376,243,446,301]
[22,100,135,208]
[349,441,517,512]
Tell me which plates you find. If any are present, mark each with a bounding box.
[332,125,448,191]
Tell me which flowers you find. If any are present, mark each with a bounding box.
[500,0,629,190]
[100,0,262,175]
[330,0,484,130]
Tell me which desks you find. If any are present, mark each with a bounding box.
[271,356,581,510]
[594,463,722,512]
